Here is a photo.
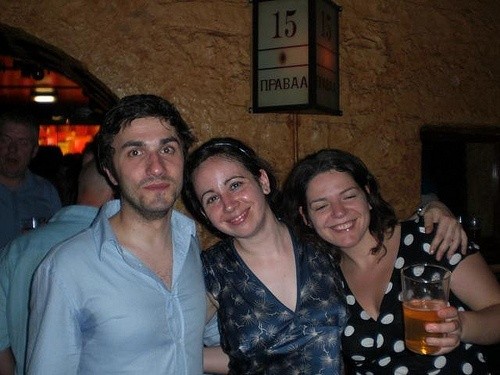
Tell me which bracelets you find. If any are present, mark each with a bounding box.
[417,209,424,218]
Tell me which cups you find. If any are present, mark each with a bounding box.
[401,264,452,354]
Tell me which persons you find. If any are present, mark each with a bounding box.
[24,94,205,375]
[181,138,467,375]
[0,107,116,375]
[202,148,500,375]
